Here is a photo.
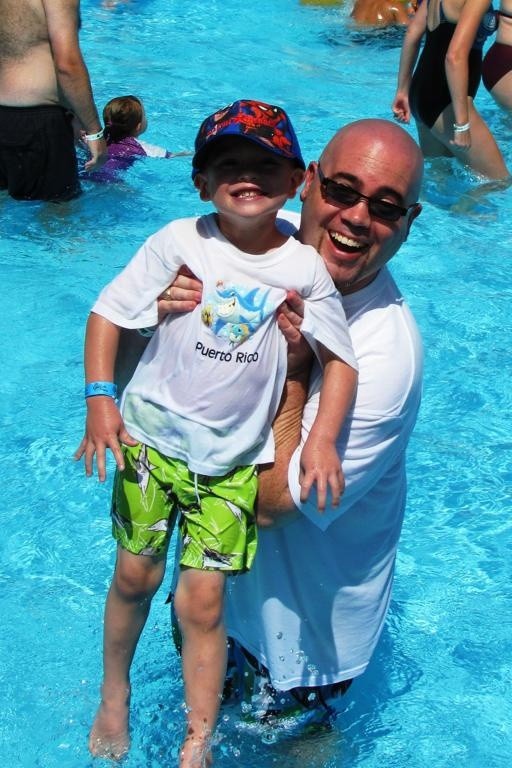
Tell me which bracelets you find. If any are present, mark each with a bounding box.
[452,121,470,133]
[84,380,119,399]
[83,127,106,142]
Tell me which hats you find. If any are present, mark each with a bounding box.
[192,100,307,174]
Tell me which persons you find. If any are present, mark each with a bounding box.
[394,0,511,220]
[112,119,442,768]
[349,0,410,30]
[481,0,511,117]
[0,1,111,205]
[32,92,197,228]
[70,97,357,767]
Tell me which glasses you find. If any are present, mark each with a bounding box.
[317,162,420,222]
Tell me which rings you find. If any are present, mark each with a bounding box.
[161,286,173,302]
